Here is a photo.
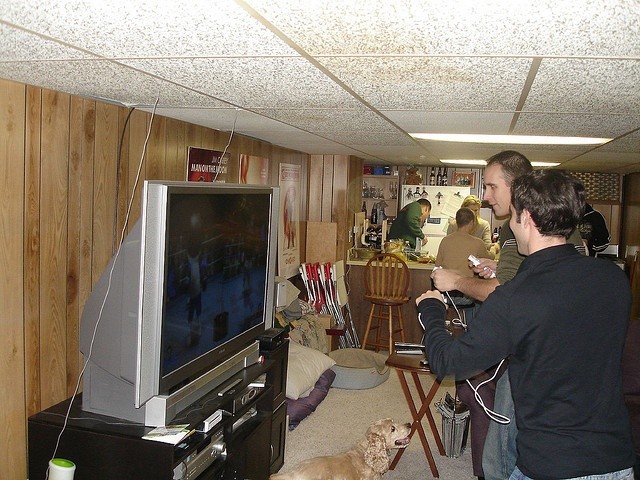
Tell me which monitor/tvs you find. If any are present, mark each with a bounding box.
[78,179,281,429]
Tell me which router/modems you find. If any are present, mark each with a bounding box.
[255,327,285,351]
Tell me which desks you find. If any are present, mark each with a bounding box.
[384,341,450,477]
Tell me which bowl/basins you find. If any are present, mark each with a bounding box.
[403,249,430,261]
[355,248,383,260]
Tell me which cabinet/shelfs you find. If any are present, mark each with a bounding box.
[360,163,491,259]
[27,339,290,480]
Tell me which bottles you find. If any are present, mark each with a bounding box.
[430,166,435,185]
[441,167,447,185]
[498,227,503,236]
[402,241,411,261]
[437,167,441,185]
[492,228,499,243]
[360,200,367,215]
[372,202,377,224]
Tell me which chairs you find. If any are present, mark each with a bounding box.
[360,254,411,353]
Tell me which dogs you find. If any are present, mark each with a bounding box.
[269,417,413,479]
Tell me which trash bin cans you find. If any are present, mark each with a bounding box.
[435,391,471,460]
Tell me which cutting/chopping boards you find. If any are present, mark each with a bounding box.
[355,211,365,258]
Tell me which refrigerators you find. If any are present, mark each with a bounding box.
[400,185,471,256]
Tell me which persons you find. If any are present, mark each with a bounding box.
[430,151,585,479]
[447,194,500,254]
[435,208,497,309]
[415,168,637,480]
[388,198,431,252]
[574,183,613,258]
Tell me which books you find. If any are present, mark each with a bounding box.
[141,423,191,445]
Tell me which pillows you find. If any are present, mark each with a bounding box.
[285,336,336,399]
[285,369,337,431]
